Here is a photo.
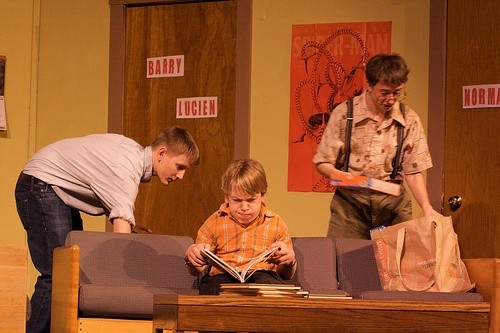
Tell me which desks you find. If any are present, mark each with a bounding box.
[153,293,491,333]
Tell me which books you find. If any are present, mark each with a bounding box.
[201,244,281,282]
[219,283,352,299]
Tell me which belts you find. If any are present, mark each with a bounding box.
[18,172,54,189]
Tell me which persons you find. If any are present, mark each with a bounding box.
[184,159,297,295]
[311,53,444,237]
[14,125,199,332]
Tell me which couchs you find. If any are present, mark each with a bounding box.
[52,230,499,333]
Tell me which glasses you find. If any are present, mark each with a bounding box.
[374,84,407,103]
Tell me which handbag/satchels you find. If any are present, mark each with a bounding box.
[370,215,476,293]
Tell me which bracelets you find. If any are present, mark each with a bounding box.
[284,260,297,268]
